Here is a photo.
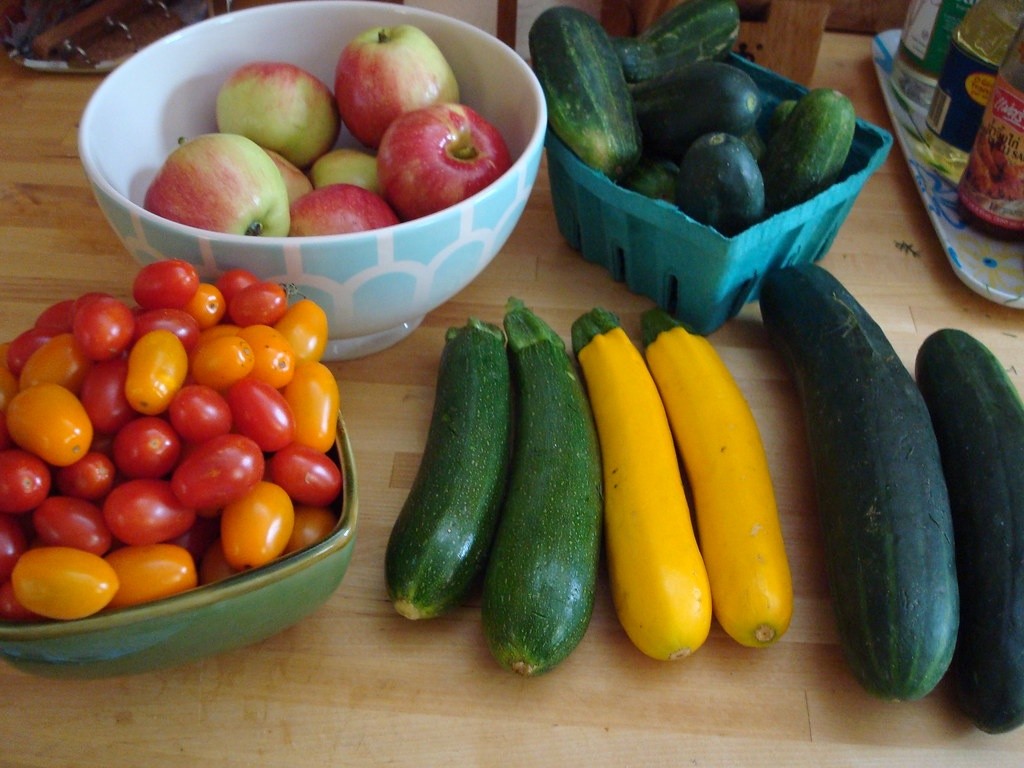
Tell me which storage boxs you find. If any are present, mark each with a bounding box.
[545,49,894,336]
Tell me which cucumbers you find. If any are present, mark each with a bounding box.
[760,263,1024,735]
[527,0,855,236]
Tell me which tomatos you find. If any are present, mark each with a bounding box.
[0,261,341,621]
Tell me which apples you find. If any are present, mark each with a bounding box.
[144,23,512,237]
